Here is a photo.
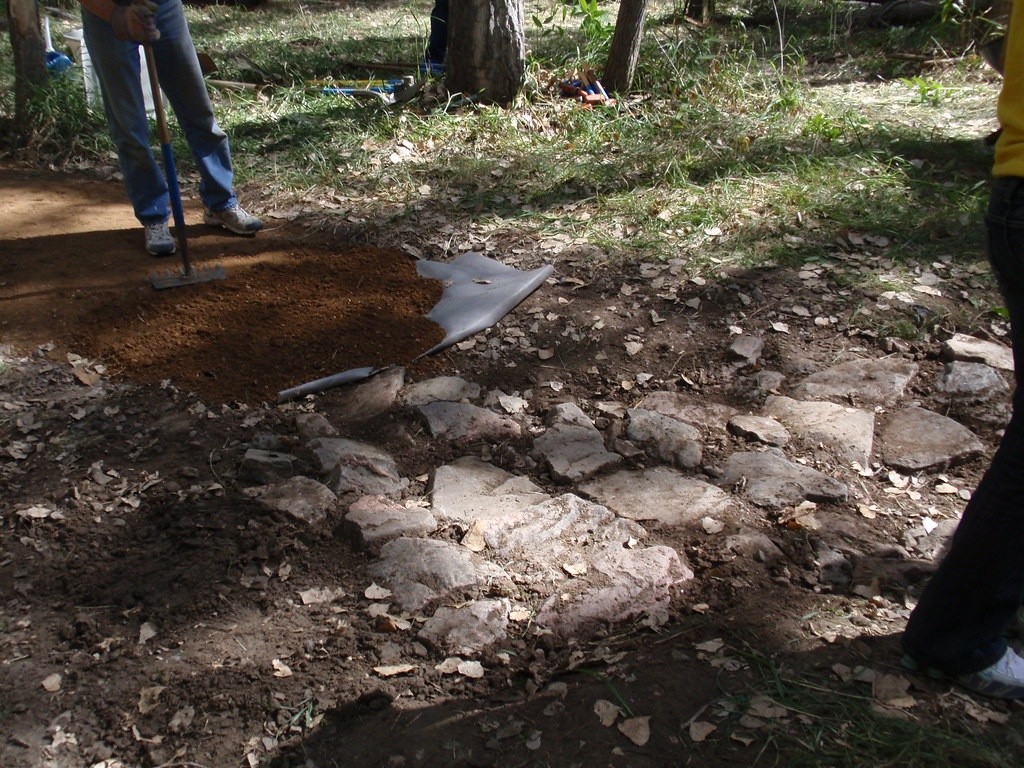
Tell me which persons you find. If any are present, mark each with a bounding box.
[79,0,263,258]
[901,0,1024,698]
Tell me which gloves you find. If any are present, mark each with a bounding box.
[111,0,160,42]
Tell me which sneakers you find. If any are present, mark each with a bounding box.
[900,635,1024,698]
[203,204,263,234]
[144,220,176,256]
